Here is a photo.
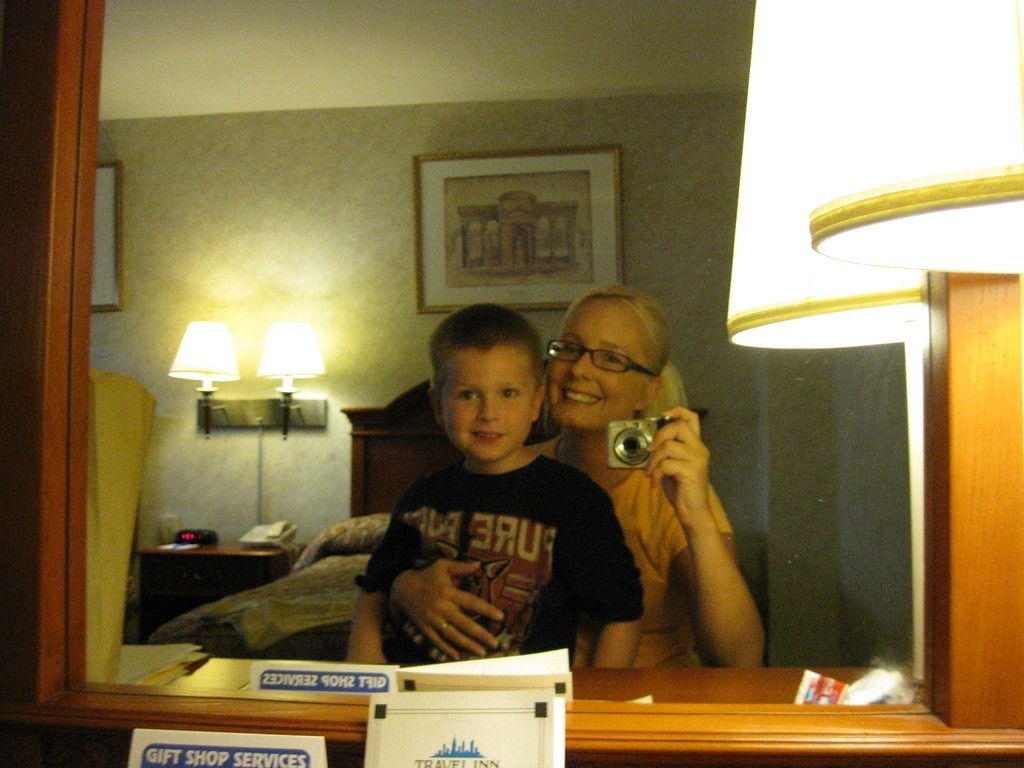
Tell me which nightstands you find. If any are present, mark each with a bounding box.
[135,542,308,645]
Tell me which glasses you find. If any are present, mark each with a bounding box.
[546,339,659,377]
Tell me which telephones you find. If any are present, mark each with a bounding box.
[239,520,298,547]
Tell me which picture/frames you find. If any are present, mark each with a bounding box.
[411,143,626,316]
[91,159,125,314]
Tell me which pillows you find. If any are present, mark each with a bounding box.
[292,512,390,570]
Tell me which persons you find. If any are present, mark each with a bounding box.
[343,304,645,670]
[388,284,766,670]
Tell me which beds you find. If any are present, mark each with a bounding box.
[144,405,711,660]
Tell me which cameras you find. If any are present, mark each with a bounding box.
[605,414,675,471]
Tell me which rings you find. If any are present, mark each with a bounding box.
[438,621,448,632]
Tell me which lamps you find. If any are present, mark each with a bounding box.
[168,320,329,442]
[777,0,1024,276]
[726,0,929,350]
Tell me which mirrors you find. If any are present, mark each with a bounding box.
[0,0,1024,768]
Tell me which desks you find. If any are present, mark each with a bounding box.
[185,661,873,704]
